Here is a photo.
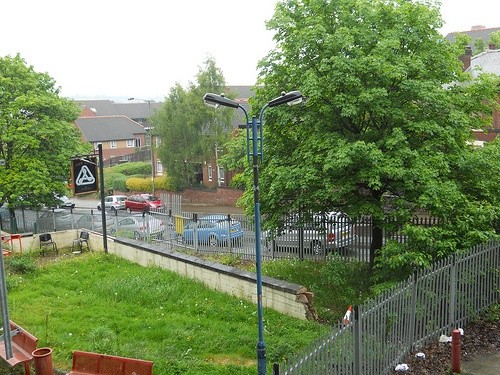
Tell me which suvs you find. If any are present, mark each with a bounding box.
[32,208,74,231]
[97,195,129,213]
[125,193,167,215]
[259,211,357,255]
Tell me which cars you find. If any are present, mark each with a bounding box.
[73,211,114,234]
[45,193,75,209]
[109,214,164,241]
[173,214,244,248]
[0,207,16,223]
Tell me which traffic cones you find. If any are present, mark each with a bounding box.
[333,305,353,332]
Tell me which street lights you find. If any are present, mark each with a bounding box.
[202,90,304,375]
[128,97,156,194]
